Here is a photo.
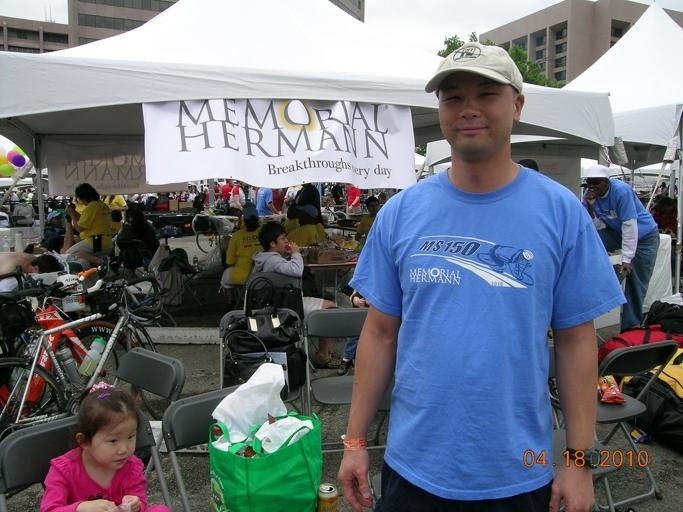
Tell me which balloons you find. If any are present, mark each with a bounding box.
[7,151,20,162]
[0,155,7,165]
[12,155,25,167]
[0,164,15,177]
[15,147,25,156]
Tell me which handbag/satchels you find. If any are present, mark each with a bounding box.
[619,347,683,455]
[598,323,683,366]
[207,409,324,512]
[251,282,301,315]
[223,276,301,353]
[639,300,683,334]
[222,328,289,402]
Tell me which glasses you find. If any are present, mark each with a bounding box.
[586,179,605,185]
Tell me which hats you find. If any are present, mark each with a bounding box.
[296,204,318,220]
[423,40,524,95]
[583,165,610,178]
[47,211,62,223]
[243,206,259,220]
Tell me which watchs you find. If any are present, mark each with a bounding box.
[564,447,602,470]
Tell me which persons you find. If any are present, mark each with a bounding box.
[128,185,209,206]
[332,183,343,204]
[281,205,300,233]
[102,194,128,223]
[13,199,34,228]
[46,213,65,234]
[582,162,659,332]
[344,183,361,213]
[25,215,73,255]
[256,188,280,216]
[0,206,13,228]
[338,287,369,376]
[116,209,160,269]
[111,209,123,234]
[336,43,627,512]
[220,207,263,306]
[214,179,255,216]
[0,187,34,202]
[355,196,380,242]
[251,221,339,369]
[0,252,62,277]
[295,183,324,242]
[41,381,170,512]
[281,186,303,214]
[324,186,332,196]
[65,183,113,266]
[287,205,319,248]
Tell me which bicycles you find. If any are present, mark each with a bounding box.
[0,266,171,438]
[325,200,347,222]
[191,205,234,253]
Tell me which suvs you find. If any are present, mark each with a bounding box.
[612,172,679,239]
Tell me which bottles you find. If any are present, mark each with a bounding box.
[318,483,338,512]
[57,343,81,383]
[79,338,106,377]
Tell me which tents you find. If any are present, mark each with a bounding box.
[426,2,682,296]
[0,0,614,242]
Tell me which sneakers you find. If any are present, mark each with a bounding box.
[102,255,112,276]
[336,358,353,376]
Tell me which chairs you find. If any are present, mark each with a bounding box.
[0,405,172,512]
[594,339,680,510]
[132,238,205,307]
[161,383,244,512]
[303,306,387,454]
[243,271,303,312]
[13,215,31,228]
[112,346,186,479]
[218,307,306,417]
[548,346,621,512]
